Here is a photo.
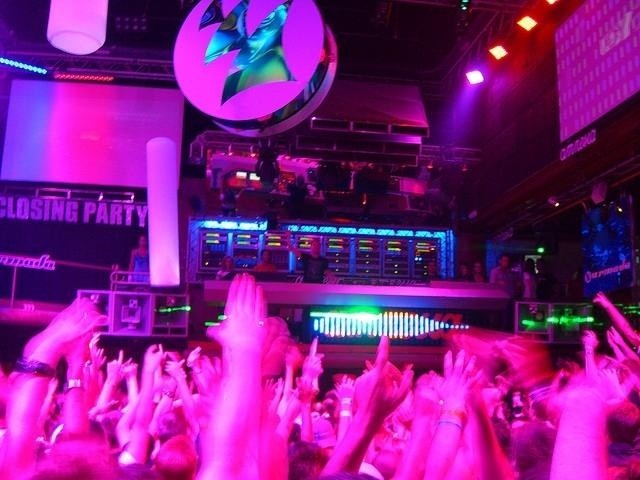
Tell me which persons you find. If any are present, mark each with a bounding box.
[0,273,640,480]
[249,250,280,280]
[459,255,556,301]
[216,255,240,280]
[286,236,338,283]
